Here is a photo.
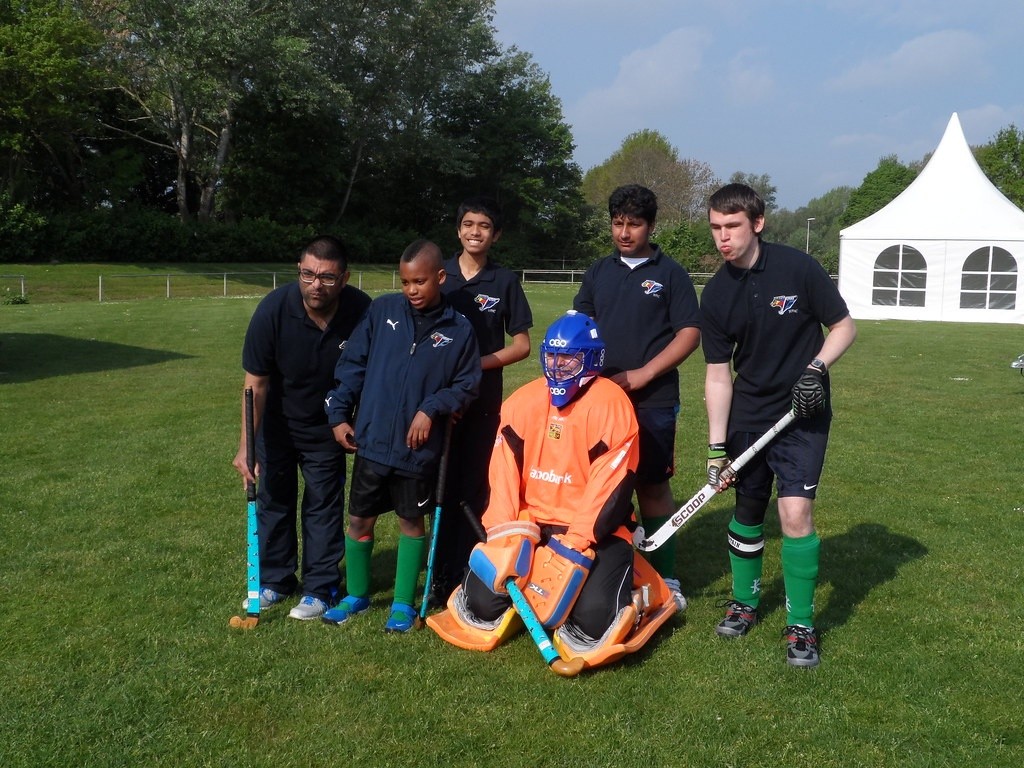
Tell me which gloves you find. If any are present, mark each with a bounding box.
[793,369,826,418]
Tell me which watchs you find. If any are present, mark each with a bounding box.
[810,359,826,374]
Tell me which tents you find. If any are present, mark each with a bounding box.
[839,113,1024,322]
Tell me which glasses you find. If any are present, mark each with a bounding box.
[298,266,345,286]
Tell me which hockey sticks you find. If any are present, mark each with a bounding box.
[415,415,452,629]
[229,385,261,631]
[631,409,796,552]
[459,499,586,677]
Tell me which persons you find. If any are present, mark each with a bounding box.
[434,199,532,603]
[323,241,483,634]
[427,311,675,667]
[572,184,701,615]
[700,182,857,664]
[233,235,372,621]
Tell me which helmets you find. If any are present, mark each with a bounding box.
[540,309,607,406]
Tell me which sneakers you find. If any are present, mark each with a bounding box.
[781,624,819,667]
[663,577,687,612]
[323,594,370,625]
[288,596,328,620]
[242,588,290,609]
[385,603,417,634]
[715,598,761,638]
